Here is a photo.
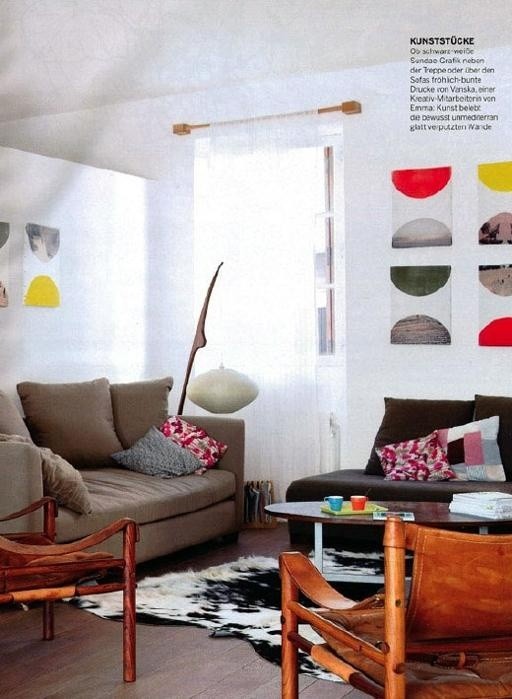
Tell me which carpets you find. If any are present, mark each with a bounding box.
[66,545,425,685]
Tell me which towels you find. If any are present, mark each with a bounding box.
[448,487,511,525]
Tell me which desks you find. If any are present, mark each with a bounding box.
[263,496,511,607]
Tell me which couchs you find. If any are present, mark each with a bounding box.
[1,415,246,578]
[283,393,510,540]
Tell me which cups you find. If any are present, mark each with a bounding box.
[324,495,343,512]
[349,495,368,511]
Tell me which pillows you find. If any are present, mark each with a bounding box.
[110,427,204,481]
[1,390,33,444]
[436,410,507,486]
[1,429,92,517]
[376,433,460,489]
[110,375,176,446]
[19,379,123,466]
[161,412,227,476]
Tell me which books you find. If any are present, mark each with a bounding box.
[373,510,415,522]
[450,491,511,520]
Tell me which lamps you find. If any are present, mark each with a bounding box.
[179,257,265,414]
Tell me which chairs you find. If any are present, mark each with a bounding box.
[1,491,139,685]
[274,515,512,699]
